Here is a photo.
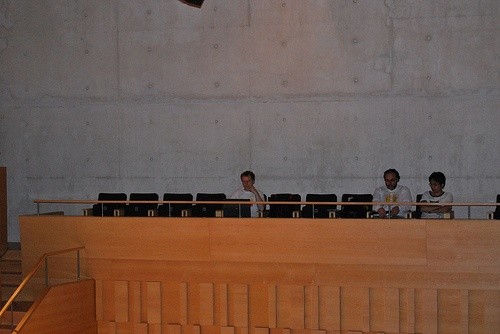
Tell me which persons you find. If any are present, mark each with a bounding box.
[419,172,453,219]
[230,171,266,218]
[372,169,413,218]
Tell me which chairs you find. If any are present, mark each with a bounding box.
[81,192,455,219]
